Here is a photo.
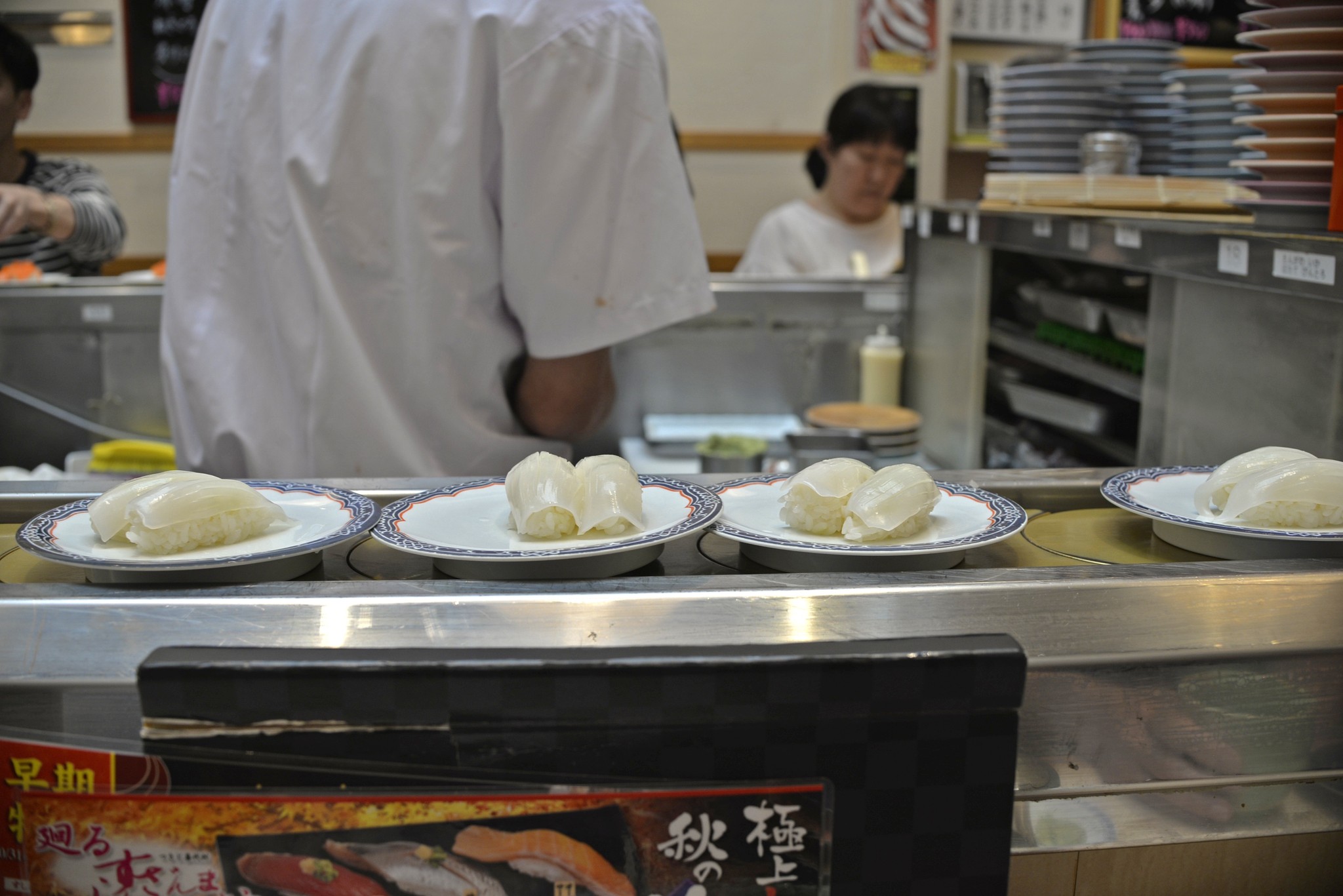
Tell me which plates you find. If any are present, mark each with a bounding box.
[642,402,921,457]
[697,471,1029,573]
[366,475,725,579]
[19,479,379,585]
[1102,464,1343,562]
[974,1,1342,235]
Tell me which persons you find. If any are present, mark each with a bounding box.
[1023,669,1244,824]
[157,1,717,479]
[730,81,927,279]
[0,24,129,277]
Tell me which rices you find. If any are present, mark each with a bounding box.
[505,452,638,538]
[778,456,941,543]
[92,469,278,557]
[1209,443,1342,528]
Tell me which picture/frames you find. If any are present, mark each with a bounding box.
[123,0,209,125]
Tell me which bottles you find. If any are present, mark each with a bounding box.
[859,324,906,404]
